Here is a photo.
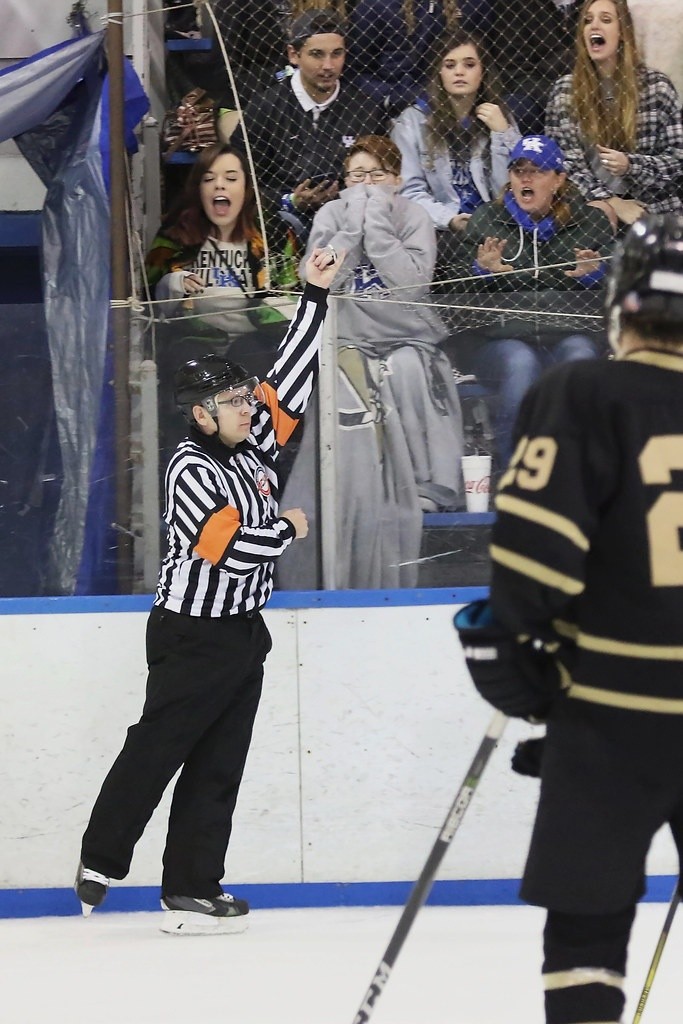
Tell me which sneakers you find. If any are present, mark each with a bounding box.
[74,858,110,917]
[160,888,249,934]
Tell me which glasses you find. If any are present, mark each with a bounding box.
[199,393,255,408]
[345,168,400,183]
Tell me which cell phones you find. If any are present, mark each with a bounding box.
[308,172,335,193]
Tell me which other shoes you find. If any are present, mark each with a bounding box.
[418,494,439,513]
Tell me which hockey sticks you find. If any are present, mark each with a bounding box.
[352,710,511,1024]
[633,878,683,1024]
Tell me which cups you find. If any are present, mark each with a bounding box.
[461,455,492,513]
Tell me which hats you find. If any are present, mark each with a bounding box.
[507,134,563,174]
[288,9,346,45]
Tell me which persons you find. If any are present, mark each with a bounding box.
[455,207,683,1024]
[141,0,683,589]
[75,253,347,933]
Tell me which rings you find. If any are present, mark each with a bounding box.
[603,161,608,165]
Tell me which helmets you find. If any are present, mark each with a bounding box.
[172,353,266,425]
[604,213,683,306]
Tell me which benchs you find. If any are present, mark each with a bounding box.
[162,39,495,528]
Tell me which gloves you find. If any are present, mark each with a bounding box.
[453,598,573,725]
